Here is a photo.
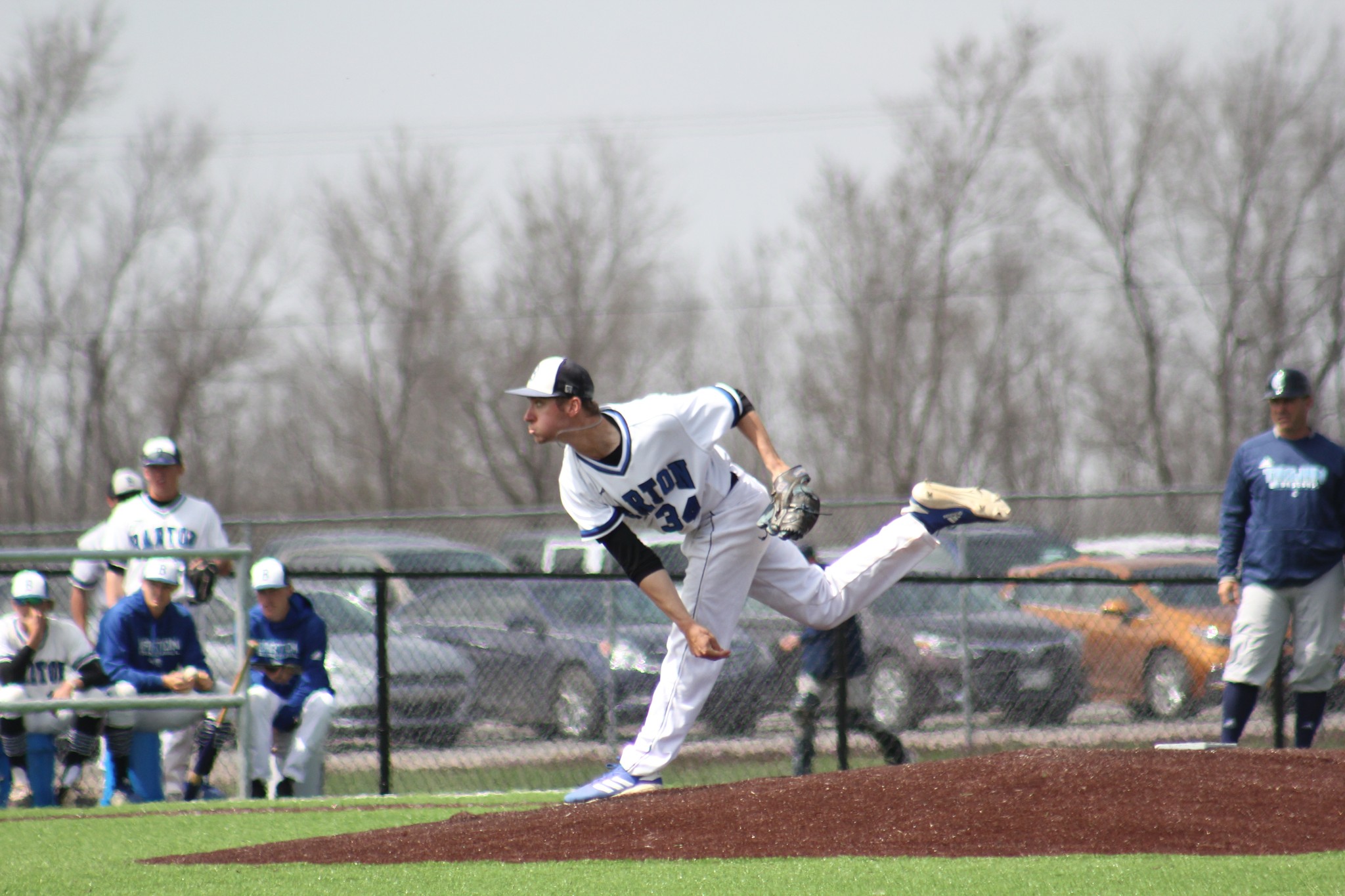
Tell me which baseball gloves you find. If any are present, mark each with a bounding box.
[184,554,222,604]
[754,464,822,543]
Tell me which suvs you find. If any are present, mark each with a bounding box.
[940,523,1080,588]
[272,538,521,616]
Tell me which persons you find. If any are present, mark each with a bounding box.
[1216,367,1344,750]
[503,354,1012,804]
[1,437,337,809]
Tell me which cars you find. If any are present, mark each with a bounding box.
[991,555,1293,709]
[0,569,466,751]
[802,546,1083,733]
[400,583,796,741]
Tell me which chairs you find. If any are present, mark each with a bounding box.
[97,731,188,805]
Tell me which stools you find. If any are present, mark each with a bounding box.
[0,732,56,808]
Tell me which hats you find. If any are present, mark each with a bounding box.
[505,357,594,401]
[109,470,143,497]
[11,569,50,600]
[141,436,181,466]
[143,556,177,586]
[1263,369,1311,399]
[250,557,290,589]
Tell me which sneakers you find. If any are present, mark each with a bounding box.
[900,482,1010,525]
[563,763,663,804]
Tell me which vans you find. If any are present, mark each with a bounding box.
[538,533,688,579]
